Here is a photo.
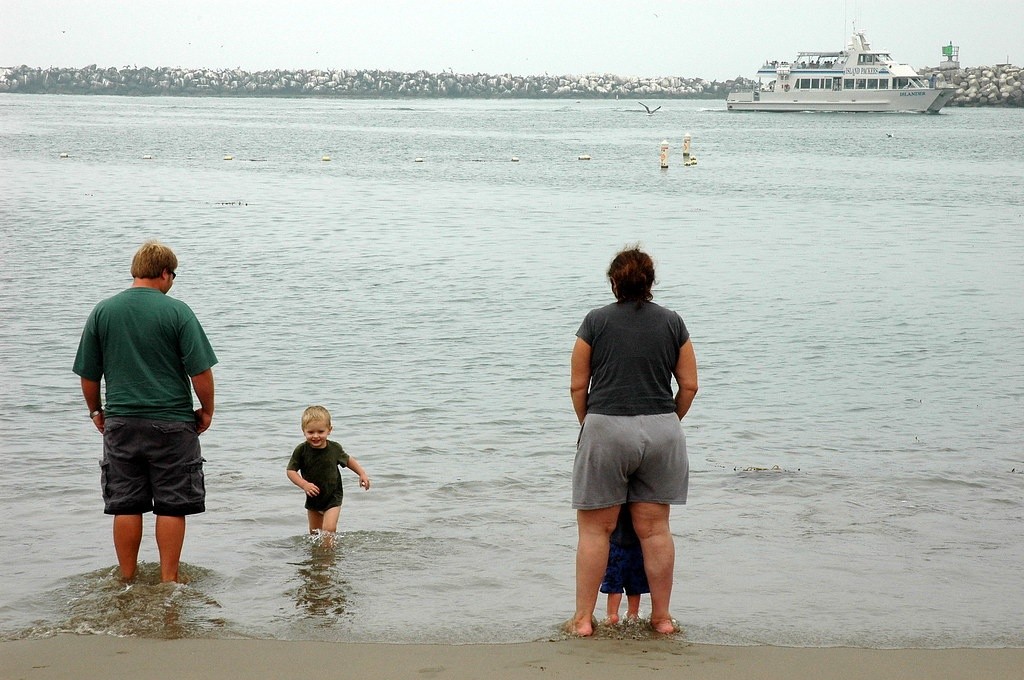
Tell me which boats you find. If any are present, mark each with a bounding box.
[727,0,964,115]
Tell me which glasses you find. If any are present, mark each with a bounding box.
[171,271,176,280]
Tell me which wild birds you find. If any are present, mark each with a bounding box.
[638,102,661,115]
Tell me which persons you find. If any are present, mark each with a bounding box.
[771,59,837,69]
[286,405,370,552]
[72,242,218,583]
[559,242,698,636]
[600,491,650,623]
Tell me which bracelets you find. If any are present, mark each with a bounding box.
[90,407,103,419]
